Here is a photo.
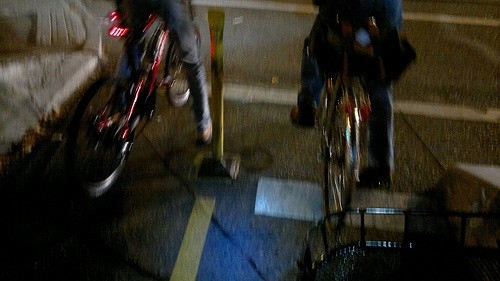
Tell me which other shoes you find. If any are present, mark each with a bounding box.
[192,118,215,145]
[98,104,123,130]
[289,102,316,128]
[373,153,396,189]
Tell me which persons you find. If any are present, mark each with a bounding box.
[290,0,418,189]
[110,1,214,143]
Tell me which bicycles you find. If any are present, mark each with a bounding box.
[62,1,204,196]
[300,0,381,236]
